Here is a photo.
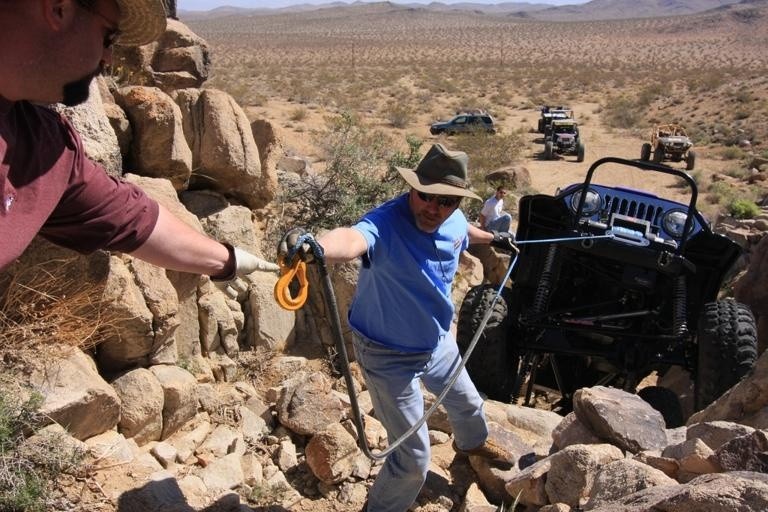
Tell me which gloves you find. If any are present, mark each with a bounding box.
[276,227,324,265]
[208,241,281,294]
[490,230,520,254]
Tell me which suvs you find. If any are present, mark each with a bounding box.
[537,104,588,161]
[456,157,757,423]
[430,110,497,137]
[639,121,699,171]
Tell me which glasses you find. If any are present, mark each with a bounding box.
[417,191,458,207]
[91,7,123,49]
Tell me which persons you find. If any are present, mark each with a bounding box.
[277,144,516,511]
[480,185,511,235]
[0,0,282,301]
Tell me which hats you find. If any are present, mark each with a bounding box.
[113,0,167,46]
[394,142,484,202]
